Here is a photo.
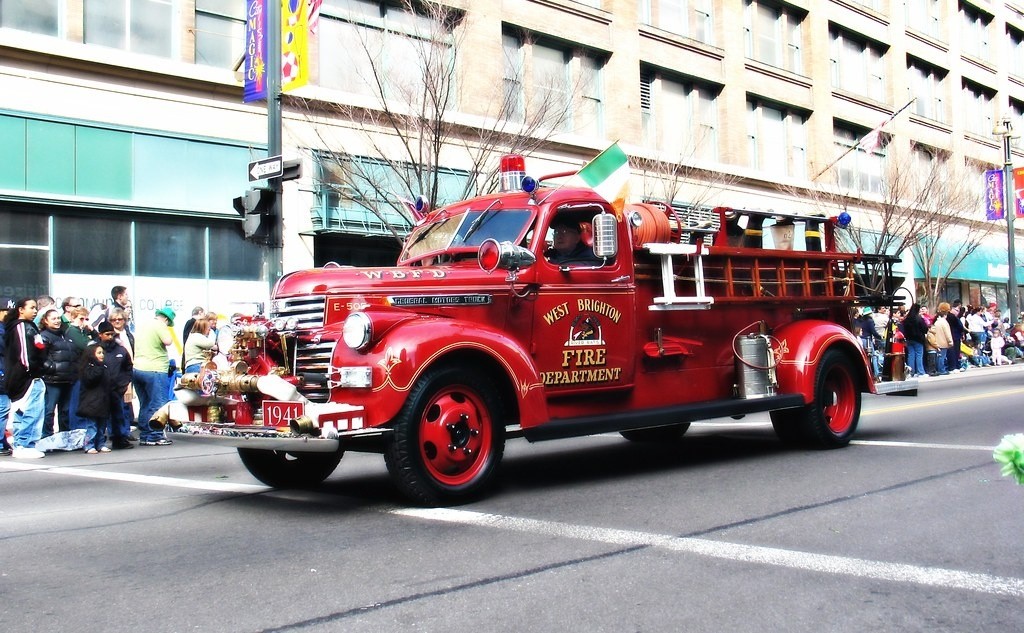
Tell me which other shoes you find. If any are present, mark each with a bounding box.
[0,449,12,456]
[986,364,991,367]
[931,371,940,375]
[960,367,965,372]
[11,445,46,459]
[112,439,134,449]
[874,376,882,383]
[101,447,111,451]
[156,439,172,445]
[87,448,98,454]
[139,440,156,445]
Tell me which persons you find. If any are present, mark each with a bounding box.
[853,300,1024,382]
[0,286,245,459]
[543,220,603,267]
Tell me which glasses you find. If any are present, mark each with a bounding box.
[551,229,574,236]
[65,305,81,308]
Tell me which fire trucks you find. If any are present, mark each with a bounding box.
[146,155,914,507]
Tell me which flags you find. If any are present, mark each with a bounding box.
[860,120,885,156]
[577,144,631,223]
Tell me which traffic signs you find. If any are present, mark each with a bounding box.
[247,153,283,181]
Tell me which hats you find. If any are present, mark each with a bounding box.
[862,306,871,316]
[155,306,176,327]
[989,301,999,306]
[938,302,951,311]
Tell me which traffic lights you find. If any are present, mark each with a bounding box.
[246,187,279,244]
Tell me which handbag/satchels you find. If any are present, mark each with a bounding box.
[895,327,905,340]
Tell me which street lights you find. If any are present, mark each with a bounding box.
[992,114,1021,329]
